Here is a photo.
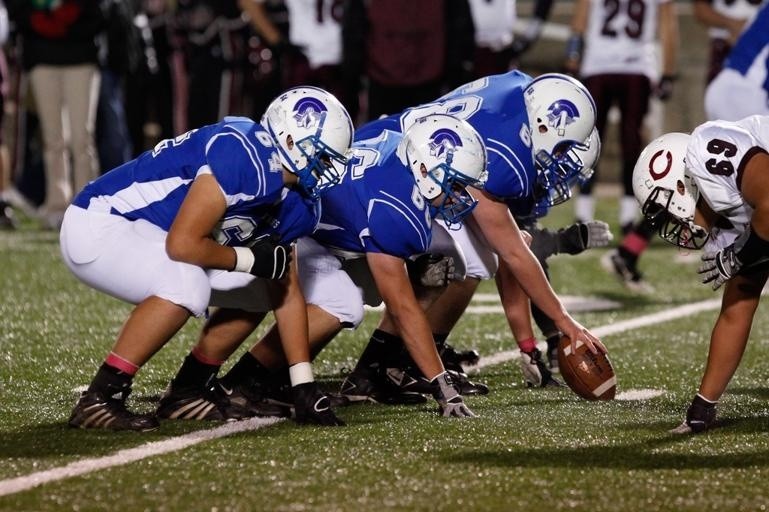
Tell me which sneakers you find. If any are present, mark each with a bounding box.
[67,386,158,434]
[599,245,654,295]
[335,345,489,407]
[156,370,346,420]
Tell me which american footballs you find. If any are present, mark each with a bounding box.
[558,335,616,400]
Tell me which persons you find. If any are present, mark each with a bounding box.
[337,71,605,407]
[212,115,491,417]
[444,125,616,369]
[58,84,357,431]
[0,0,769,299]
[630,114,767,437]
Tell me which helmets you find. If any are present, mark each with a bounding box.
[522,73,597,174]
[259,86,353,195]
[633,133,711,249]
[396,113,488,228]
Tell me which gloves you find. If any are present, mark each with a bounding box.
[516,342,560,387]
[428,371,479,418]
[545,334,562,371]
[555,220,611,255]
[227,236,293,282]
[286,382,346,427]
[411,253,455,289]
[667,397,718,434]
[698,225,766,291]
